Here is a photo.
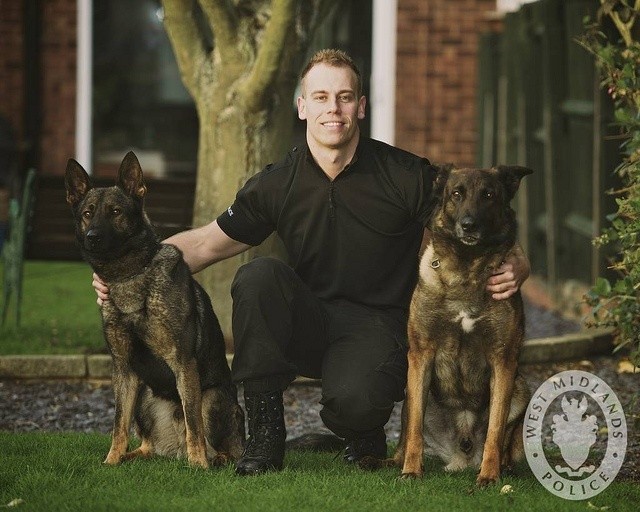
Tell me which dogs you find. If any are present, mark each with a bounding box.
[64,150,248,471]
[359,161,533,489]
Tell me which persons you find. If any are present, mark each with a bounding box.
[91,46,530,479]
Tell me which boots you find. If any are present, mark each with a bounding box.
[236,392,286,476]
[345,431,386,463]
